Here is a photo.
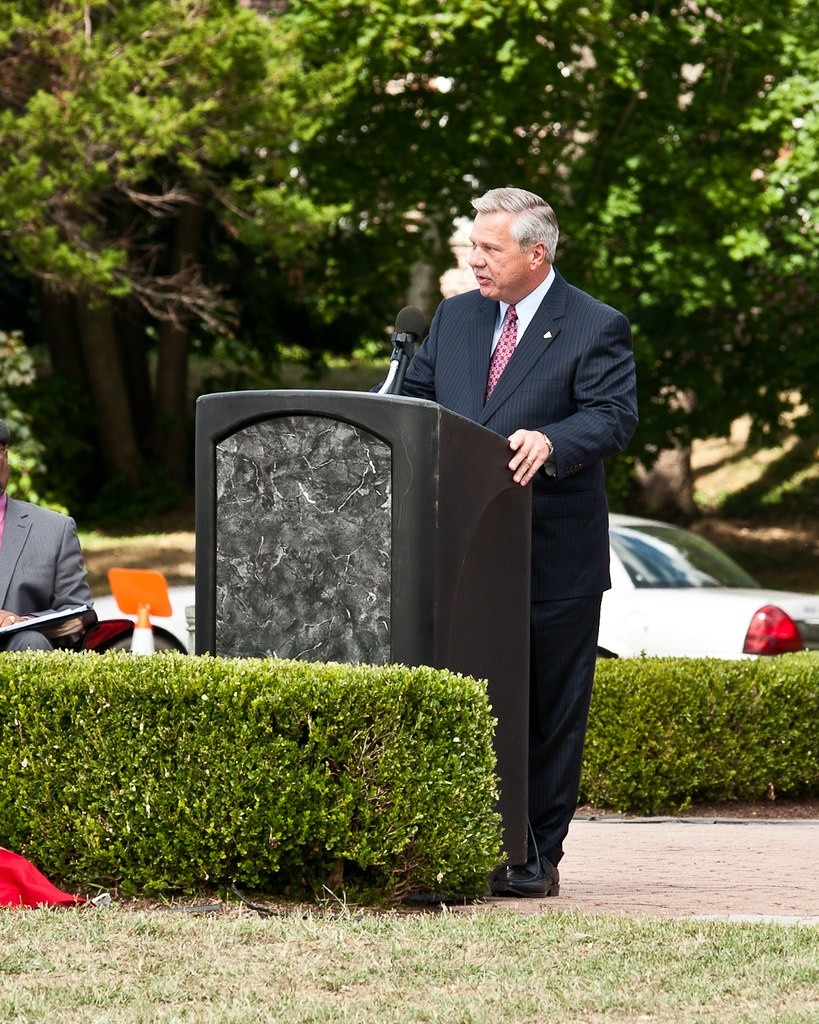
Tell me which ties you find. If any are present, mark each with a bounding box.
[485,303,518,402]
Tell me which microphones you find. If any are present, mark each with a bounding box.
[390,305,427,396]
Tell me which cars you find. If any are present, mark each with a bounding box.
[83,513,819,659]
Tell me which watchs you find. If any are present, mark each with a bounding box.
[545,434,553,457]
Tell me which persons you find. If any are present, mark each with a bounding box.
[0,422,95,652]
[366,186,640,899]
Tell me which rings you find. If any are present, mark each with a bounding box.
[7,616,16,624]
[524,458,533,464]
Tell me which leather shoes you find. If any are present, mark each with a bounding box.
[506,855,560,899]
